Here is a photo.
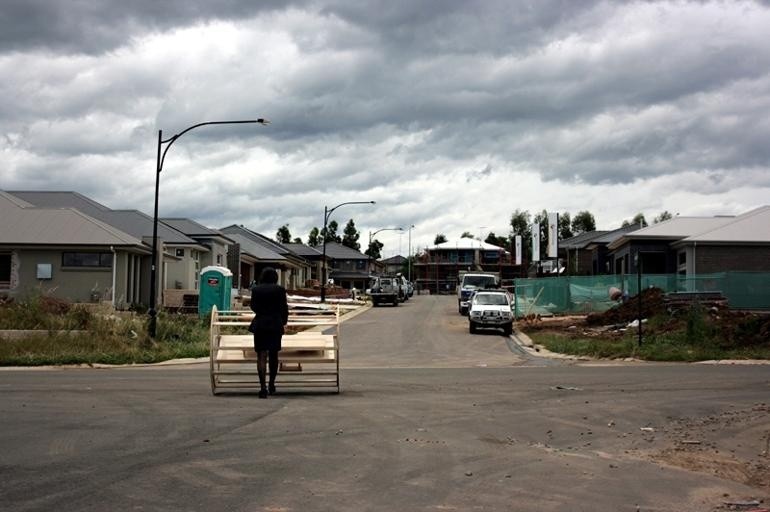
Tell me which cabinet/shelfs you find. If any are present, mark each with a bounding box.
[210,302,341,395]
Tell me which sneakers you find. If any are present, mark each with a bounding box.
[258,391,267,398]
[269,386,275,394]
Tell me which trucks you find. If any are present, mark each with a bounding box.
[366,273,414,306]
[456,274,498,313]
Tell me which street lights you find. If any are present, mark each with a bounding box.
[320,201,374,302]
[146,119,271,340]
[362,228,402,297]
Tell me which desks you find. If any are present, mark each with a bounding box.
[163,289,199,309]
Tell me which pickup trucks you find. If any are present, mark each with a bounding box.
[468,289,513,334]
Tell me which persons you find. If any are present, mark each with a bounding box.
[417,282,422,296]
[249,267,289,397]
[445,283,450,297]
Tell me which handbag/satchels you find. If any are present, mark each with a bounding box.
[248,317,255,333]
